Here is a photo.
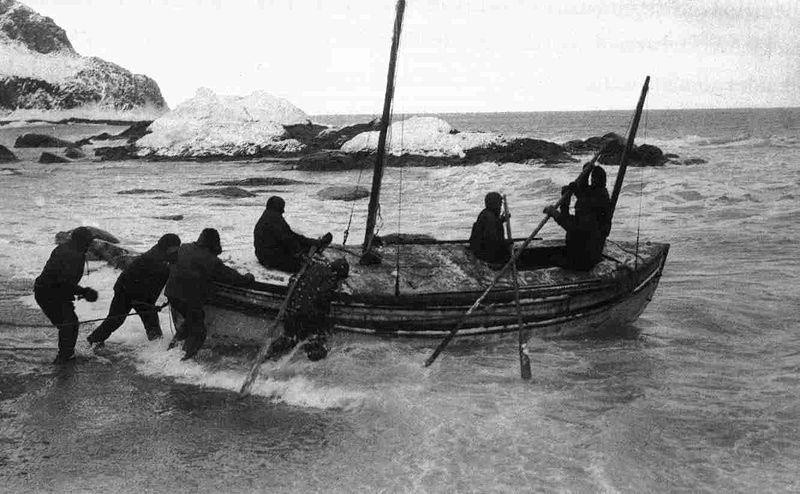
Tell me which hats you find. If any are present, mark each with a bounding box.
[196,228,222,254]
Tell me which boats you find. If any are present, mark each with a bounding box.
[54,225,671,353]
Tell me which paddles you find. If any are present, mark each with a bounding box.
[239,240,317,398]
[423,150,604,367]
[503,195,531,379]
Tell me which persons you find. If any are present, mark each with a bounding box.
[543,161,612,271]
[87,233,181,349]
[260,258,350,361]
[253,196,333,274]
[34,227,98,362]
[164,227,255,361]
[470,192,514,265]
